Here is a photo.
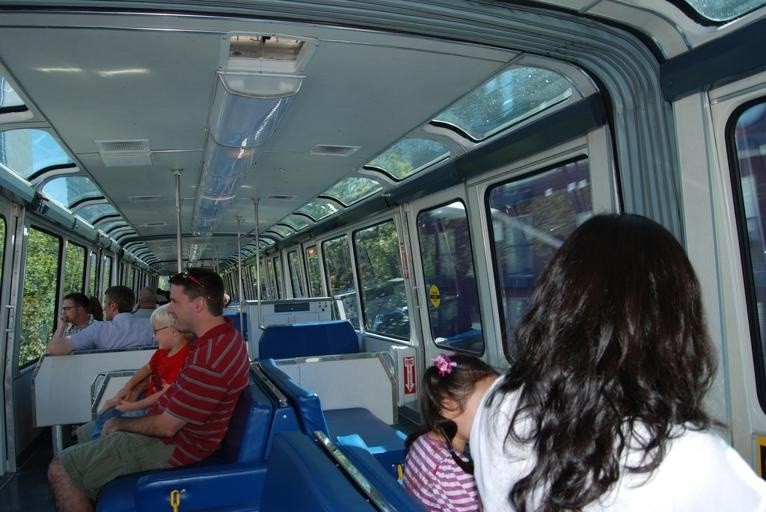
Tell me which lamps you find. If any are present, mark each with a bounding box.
[186,70,306,271]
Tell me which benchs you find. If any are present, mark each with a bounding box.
[334,433,432,512]
[430,306,482,349]
[93,370,273,512]
[258,352,399,428]
[31,348,224,455]
[135,431,378,512]
[258,320,363,357]
[252,296,362,358]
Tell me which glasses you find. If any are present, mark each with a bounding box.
[182,268,211,300]
[62,306,78,310]
[153,326,169,334]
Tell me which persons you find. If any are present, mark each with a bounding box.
[53,292,98,339]
[87,295,103,321]
[469,212,766,512]
[47,285,158,355]
[157,288,170,303]
[47,267,250,512]
[132,286,157,317]
[401,352,502,511]
[84,300,192,443]
